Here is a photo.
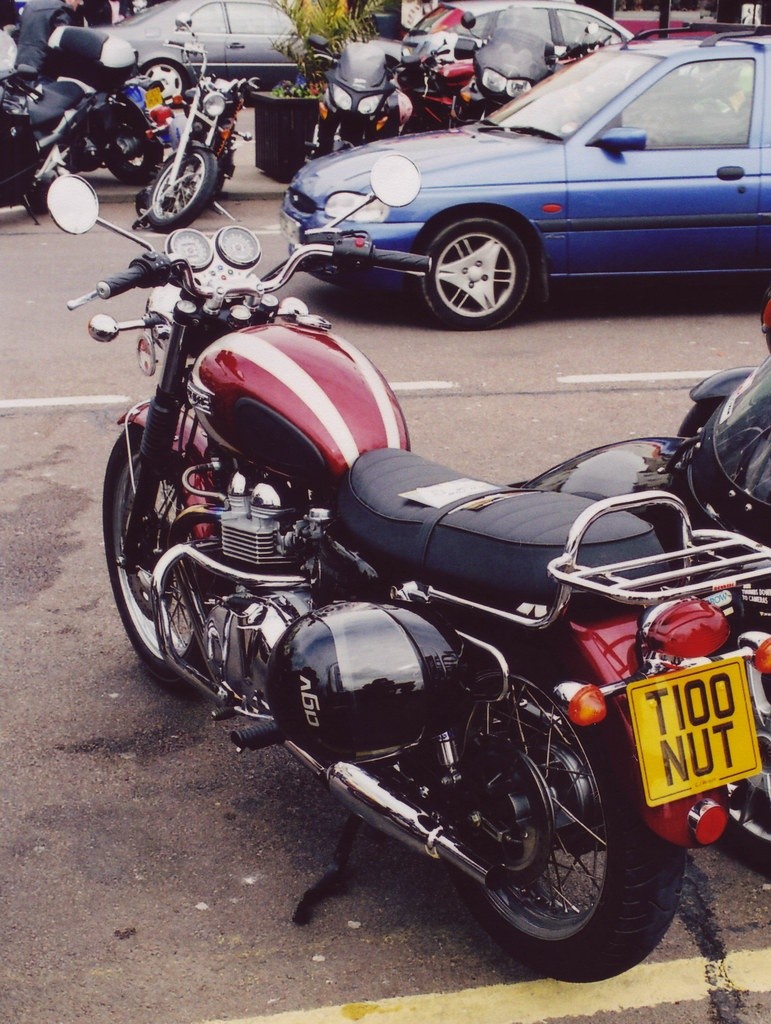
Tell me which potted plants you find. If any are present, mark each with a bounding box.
[251,80,320,175]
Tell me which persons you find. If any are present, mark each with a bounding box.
[83,0,124,27]
[15,0,84,78]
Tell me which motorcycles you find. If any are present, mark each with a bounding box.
[496,305,770,890]
[43,174,770,985]
[291,0,770,183]
[133,8,262,234]
[1,19,181,230]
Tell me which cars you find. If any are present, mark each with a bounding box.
[280,25,770,332]
[109,0,305,106]
